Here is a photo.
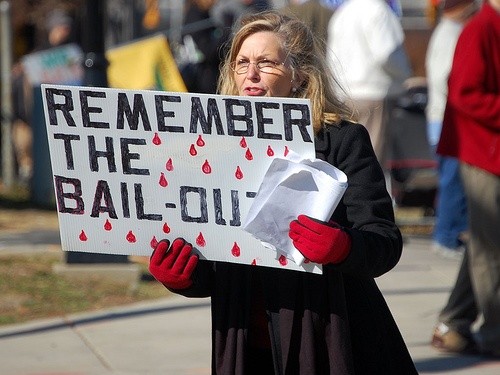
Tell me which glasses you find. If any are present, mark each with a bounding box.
[228,59,290,72]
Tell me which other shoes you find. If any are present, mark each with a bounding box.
[432,327,474,353]
[429,239,465,260]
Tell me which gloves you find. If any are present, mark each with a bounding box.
[149,237,199,290]
[289,215,352,265]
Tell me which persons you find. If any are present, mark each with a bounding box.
[430,0,500,360]
[149,10,419,375]
[424,0,480,259]
[13,10,87,212]
[108,0,274,94]
[325,0,413,203]
